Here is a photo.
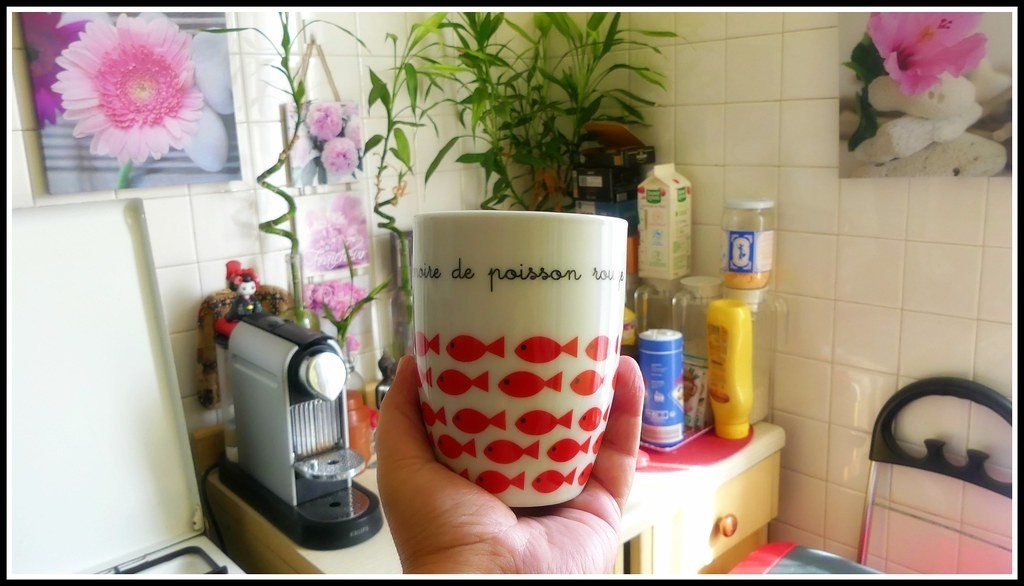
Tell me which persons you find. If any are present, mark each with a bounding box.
[372,353,646,574]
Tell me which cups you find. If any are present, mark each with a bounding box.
[411,210,629,509]
[671,275,723,357]
[632,277,682,335]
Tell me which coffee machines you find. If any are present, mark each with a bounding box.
[213,314,385,553]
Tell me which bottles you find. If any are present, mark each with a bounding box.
[718,199,778,287]
[706,299,753,440]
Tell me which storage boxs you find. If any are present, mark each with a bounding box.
[571,147,656,272]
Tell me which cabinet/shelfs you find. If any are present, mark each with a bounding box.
[205,421,787,574]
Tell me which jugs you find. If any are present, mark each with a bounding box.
[720,286,791,425]
